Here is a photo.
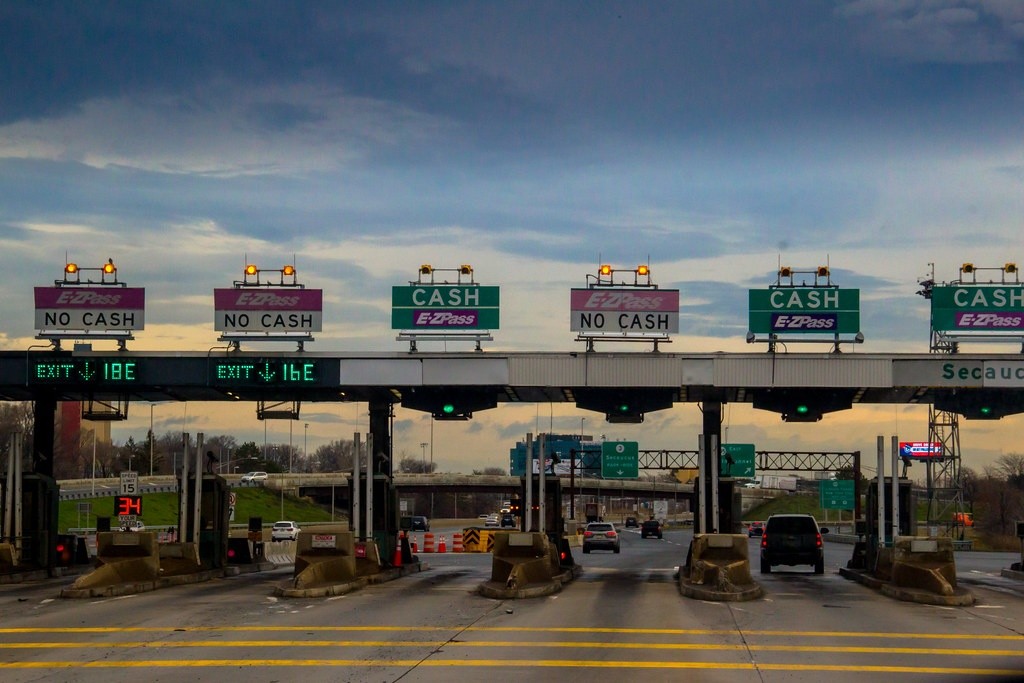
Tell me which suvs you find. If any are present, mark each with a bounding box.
[242,470,269,482]
[270,520,302,542]
[760,513,830,573]
[577,522,622,553]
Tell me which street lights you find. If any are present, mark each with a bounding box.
[304,423,310,455]
[581,416,586,479]
[420,442,428,473]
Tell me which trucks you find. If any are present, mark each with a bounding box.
[743,475,799,491]
[585,503,606,521]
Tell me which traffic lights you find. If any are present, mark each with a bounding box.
[787,401,820,417]
[435,399,463,414]
[969,401,1000,417]
[608,401,639,417]
[510,498,521,513]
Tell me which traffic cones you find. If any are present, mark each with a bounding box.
[437,536,447,553]
[390,537,405,568]
[413,535,418,552]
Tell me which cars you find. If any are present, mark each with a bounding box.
[638,520,665,538]
[626,517,639,528]
[411,515,431,532]
[478,502,516,528]
[749,521,764,537]
[118,520,146,532]
